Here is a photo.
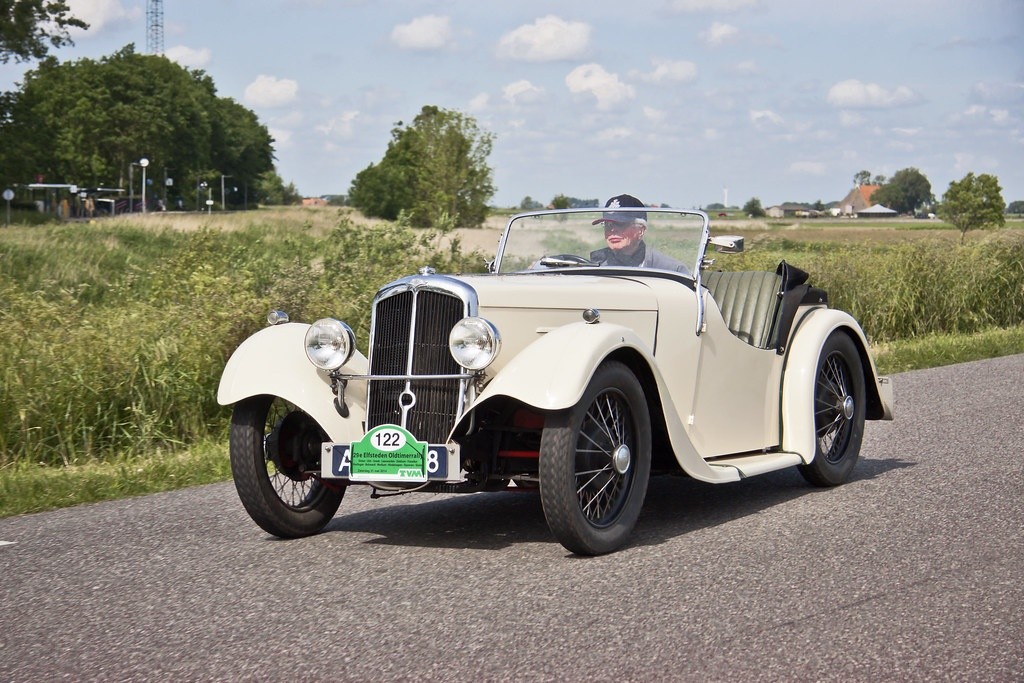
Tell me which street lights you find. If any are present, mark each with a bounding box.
[222,175,232,210]
[129,162,138,213]
[140,158,149,214]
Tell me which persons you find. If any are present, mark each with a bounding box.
[591,194,693,277]
[57,190,95,220]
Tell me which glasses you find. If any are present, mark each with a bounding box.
[601,221,639,230]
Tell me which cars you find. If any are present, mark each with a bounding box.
[218,207,895,556]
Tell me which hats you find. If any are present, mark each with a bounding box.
[592,194,647,226]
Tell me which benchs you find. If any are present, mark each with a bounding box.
[689,268,784,349]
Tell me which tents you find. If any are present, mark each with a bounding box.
[856,203,898,218]
